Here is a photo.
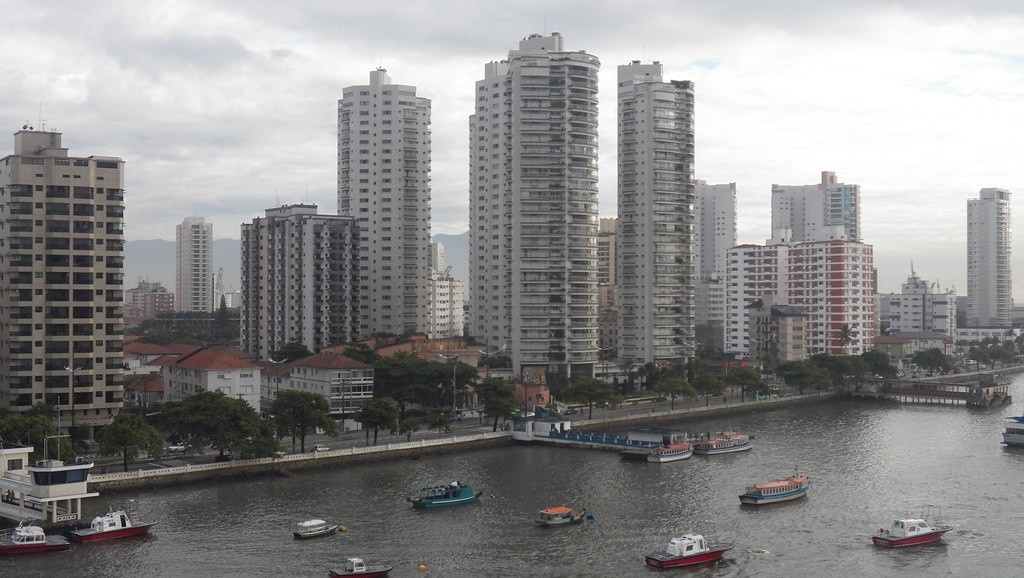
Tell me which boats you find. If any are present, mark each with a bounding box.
[694,430,753,454]
[738,463,813,505]
[870,505,954,548]
[328,555,394,578]
[293,519,338,538]
[647,441,695,462]
[0,524,71,554]
[533,504,586,526]
[70,509,159,543]
[406,481,484,507]
[999,412,1024,447]
[644,533,735,568]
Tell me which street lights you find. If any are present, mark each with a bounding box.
[63,364,86,449]
[266,356,290,439]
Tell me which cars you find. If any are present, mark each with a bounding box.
[169,443,193,452]
[315,445,331,451]
